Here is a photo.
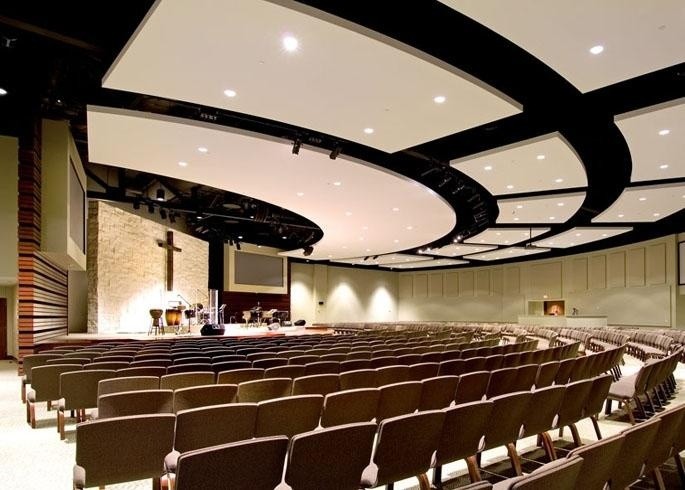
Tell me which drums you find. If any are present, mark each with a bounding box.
[150,310,163,326]
[165,309,182,326]
[199,313,210,319]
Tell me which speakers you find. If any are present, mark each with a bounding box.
[294,320,305,326]
[201,324,225,335]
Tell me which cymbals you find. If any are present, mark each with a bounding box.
[193,303,203,309]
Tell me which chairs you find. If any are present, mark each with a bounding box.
[282,422,378,490]
[536,378,593,448]
[476,391,531,479]
[18,317,685,426]
[255,394,323,437]
[159,435,289,490]
[433,401,484,490]
[73,413,175,489]
[362,411,445,490]
[26,364,84,428]
[57,370,117,439]
[559,374,612,440]
[174,402,258,453]
[453,402,684,490]
[507,385,566,462]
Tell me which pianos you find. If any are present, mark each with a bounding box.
[242,309,289,328]
